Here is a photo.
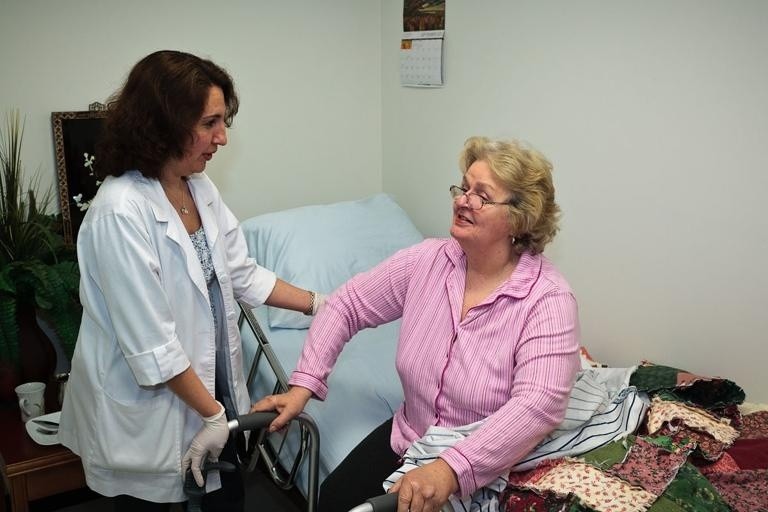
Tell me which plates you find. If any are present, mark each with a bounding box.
[25,411,61,446]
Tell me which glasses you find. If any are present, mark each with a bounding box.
[449,184,510,211]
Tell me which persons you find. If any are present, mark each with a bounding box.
[249,135,583,512]
[57,50,329,512]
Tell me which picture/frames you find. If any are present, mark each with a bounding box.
[52,109,119,248]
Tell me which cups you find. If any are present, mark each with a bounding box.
[13,381,46,422]
[55,373,69,407]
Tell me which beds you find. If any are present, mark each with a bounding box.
[227,201,768,510]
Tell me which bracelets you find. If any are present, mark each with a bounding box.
[304,291,315,316]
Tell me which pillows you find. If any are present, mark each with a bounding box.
[242,193,425,329]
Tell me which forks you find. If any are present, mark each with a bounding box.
[38,428,57,435]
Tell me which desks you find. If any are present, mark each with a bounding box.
[0,389,88,511]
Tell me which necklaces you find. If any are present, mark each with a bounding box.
[167,190,191,215]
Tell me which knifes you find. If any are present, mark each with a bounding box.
[32,418,58,428]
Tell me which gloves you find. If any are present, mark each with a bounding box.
[181,399,230,487]
[311,290,328,315]
